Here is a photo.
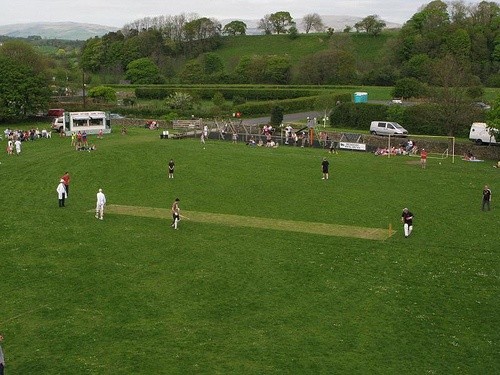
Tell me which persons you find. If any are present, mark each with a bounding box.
[401,208,414,238]
[14,138,21,156]
[62,172,70,196]
[202,124,209,140]
[7,139,13,155]
[71,112,110,151]
[480,186,493,210]
[0,127,52,142]
[321,158,329,180]
[56,179,67,208]
[421,148,428,169]
[200,133,205,144]
[167,159,175,179]
[171,198,181,231]
[95,189,106,220]
[378,139,417,156]
[82,131,88,146]
[77,131,82,146]
[231,123,340,154]
[468,150,476,160]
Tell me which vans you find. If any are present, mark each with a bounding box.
[370,121,409,136]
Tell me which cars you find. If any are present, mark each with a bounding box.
[51,117,63,133]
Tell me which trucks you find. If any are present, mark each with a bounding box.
[469,122,500,145]
